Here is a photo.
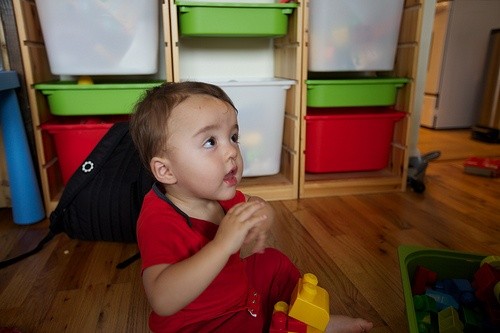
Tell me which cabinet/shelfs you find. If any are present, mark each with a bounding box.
[12,0,436,217]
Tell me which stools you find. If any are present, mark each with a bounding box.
[0,70,45,225]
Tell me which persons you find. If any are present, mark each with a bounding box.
[131,81,374,333]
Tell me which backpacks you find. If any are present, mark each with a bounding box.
[49,122,157,243]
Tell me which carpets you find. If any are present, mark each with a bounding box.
[398,245,500,333]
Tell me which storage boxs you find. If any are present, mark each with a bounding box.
[207,76,296,177]
[175,3,298,39]
[32,1,167,194]
[306,79,411,173]
